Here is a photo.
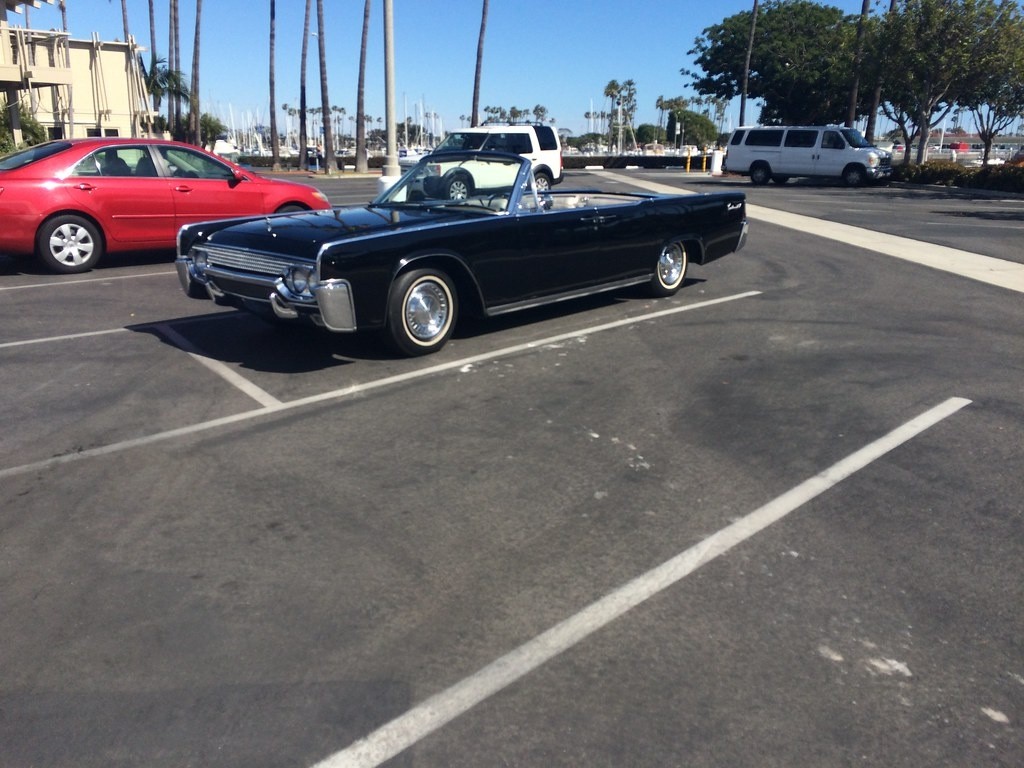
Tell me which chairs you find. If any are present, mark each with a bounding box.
[135,156,159,177]
[96,156,132,177]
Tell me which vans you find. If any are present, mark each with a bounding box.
[720,121,894,188]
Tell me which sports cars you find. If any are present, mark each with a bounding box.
[175,143,750,356]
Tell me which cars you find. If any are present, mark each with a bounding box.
[233,143,436,158]
[872,141,1024,165]
[561,141,718,158]
[1,137,336,276]
[397,147,435,159]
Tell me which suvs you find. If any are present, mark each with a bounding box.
[381,121,564,201]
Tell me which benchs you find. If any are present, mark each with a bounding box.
[520,194,641,211]
